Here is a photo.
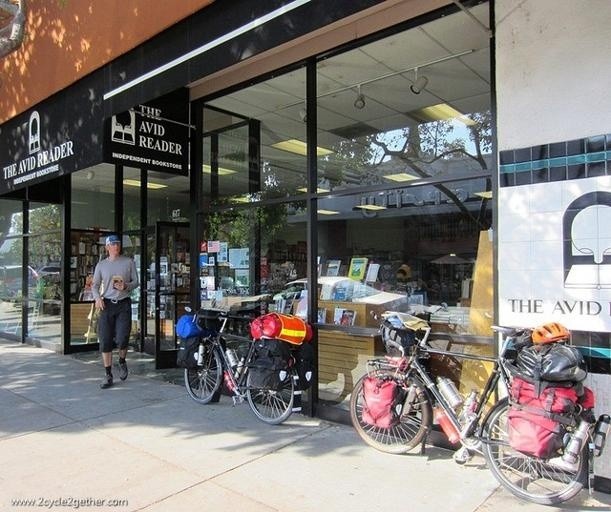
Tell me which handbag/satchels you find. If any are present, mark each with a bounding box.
[512,375,594,412]
[293,342,312,390]
[246,339,290,390]
[507,407,563,459]
[518,344,588,382]
[177,337,226,370]
[362,378,397,428]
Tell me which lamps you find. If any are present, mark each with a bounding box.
[297,66,428,124]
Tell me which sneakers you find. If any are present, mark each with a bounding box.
[100,374,113,388]
[118,361,127,380]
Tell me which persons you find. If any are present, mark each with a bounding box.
[91,235,139,390]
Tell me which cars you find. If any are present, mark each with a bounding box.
[281,277,406,325]
[0,266,61,302]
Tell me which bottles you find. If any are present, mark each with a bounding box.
[434,375,479,421]
[197,342,205,367]
[563,415,610,462]
[224,345,246,384]
[217,311,228,334]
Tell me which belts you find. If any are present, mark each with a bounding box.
[103,297,131,304]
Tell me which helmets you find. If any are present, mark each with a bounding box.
[380,320,416,347]
[531,324,570,343]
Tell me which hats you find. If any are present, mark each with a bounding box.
[106,235,120,246]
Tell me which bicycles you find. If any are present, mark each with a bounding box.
[184,306,295,425]
[349,311,594,505]
